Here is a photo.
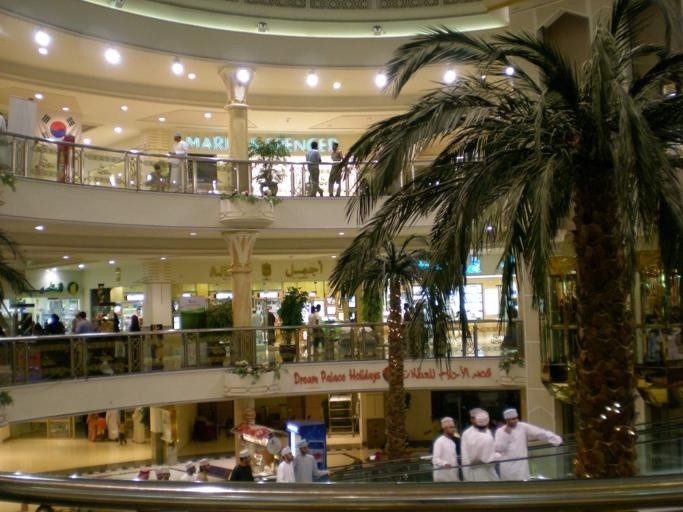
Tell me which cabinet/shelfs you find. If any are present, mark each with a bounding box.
[327,392,355,438]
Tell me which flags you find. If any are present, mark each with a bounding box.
[35,110,83,145]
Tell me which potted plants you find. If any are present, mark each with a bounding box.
[249,137,295,197]
[198,299,235,366]
[276,286,310,362]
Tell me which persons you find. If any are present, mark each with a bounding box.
[166,131,190,193]
[329,141,347,198]
[431,407,564,482]
[401,302,415,323]
[306,141,323,197]
[86,410,127,445]
[135,439,335,484]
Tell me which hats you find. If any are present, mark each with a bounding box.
[239,449,250,457]
[139,458,210,474]
[468,408,490,426]
[297,439,308,448]
[280,447,291,457]
[440,416,455,427]
[502,407,518,420]
